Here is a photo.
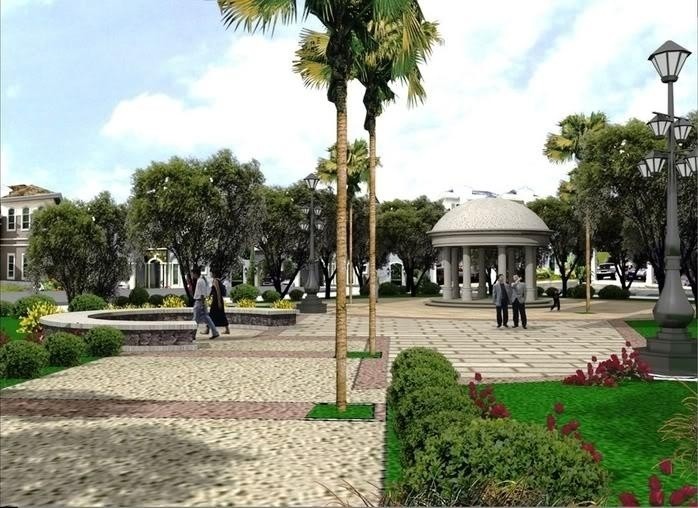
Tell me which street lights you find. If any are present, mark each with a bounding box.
[298,172,326,313]
[646,38,697,329]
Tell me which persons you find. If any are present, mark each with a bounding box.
[492,274,511,327]
[550,291,561,311]
[200,269,230,334]
[511,274,527,329]
[193,269,219,340]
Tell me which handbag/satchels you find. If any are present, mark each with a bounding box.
[212,286,226,297]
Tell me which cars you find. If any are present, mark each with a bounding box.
[596,262,616,280]
[627,266,646,281]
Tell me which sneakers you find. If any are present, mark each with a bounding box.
[200,331,209,334]
[209,334,219,339]
[497,323,529,328]
[221,331,230,334]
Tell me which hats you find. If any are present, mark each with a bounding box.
[191,267,201,274]
